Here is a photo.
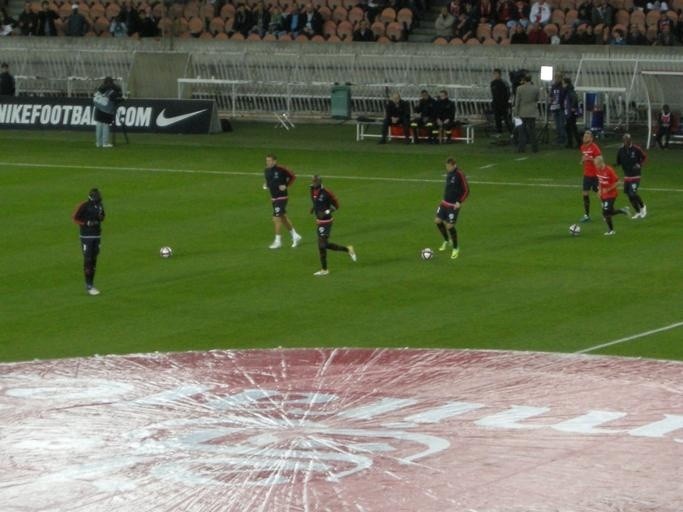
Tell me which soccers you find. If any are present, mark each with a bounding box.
[421,247,434,261]
[160,246,172,260]
[569,224,581,236]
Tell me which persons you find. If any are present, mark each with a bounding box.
[656,105,675,147]
[92,77,126,149]
[435,159,470,260]
[2,2,683,47]
[376,89,459,147]
[488,68,587,153]
[577,129,648,239]
[0,63,18,98]
[71,188,108,296]
[307,175,357,277]
[261,154,303,251]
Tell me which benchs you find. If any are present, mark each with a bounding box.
[652,119,682,149]
[353,113,475,144]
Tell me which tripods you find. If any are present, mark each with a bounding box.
[537,90,559,143]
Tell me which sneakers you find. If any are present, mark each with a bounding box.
[88,287,100,295]
[604,230,617,236]
[269,237,281,250]
[314,269,329,276]
[348,245,358,262]
[494,133,581,152]
[622,206,631,219]
[292,235,302,248]
[632,206,647,219]
[377,138,454,144]
[580,215,592,222]
[449,249,460,259]
[439,240,448,250]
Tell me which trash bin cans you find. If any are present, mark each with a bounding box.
[330,86,351,119]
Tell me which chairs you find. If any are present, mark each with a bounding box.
[19,1,682,50]
